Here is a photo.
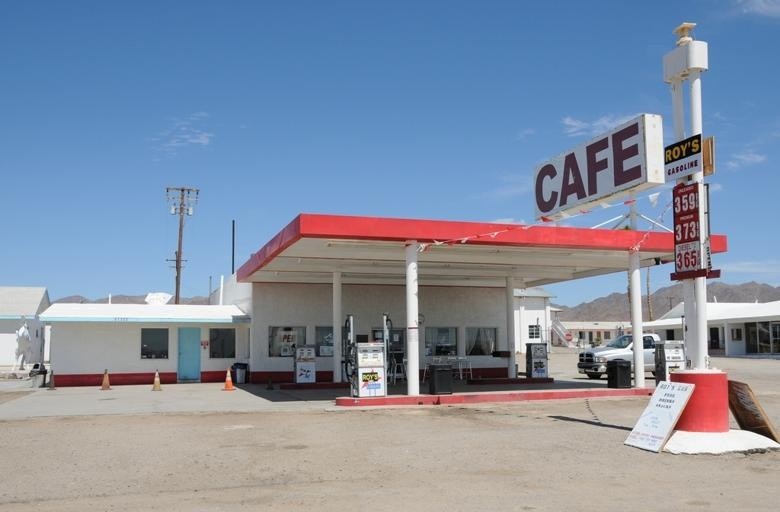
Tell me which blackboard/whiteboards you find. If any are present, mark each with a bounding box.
[623,381,696,453]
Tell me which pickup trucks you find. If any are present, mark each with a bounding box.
[578,334,684,380]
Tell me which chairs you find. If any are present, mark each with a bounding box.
[388,354,474,385]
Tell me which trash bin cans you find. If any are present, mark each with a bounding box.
[31,363,47,388]
[429,365,453,395]
[234,363,248,384]
[607,359,631,388]
